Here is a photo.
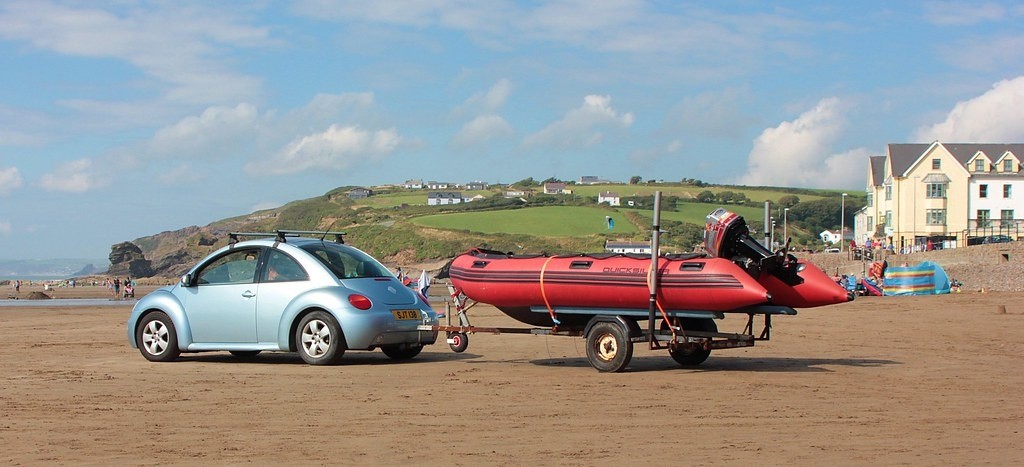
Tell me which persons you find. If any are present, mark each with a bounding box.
[30,276,134,298]
[878,239,882,246]
[831,272,840,281]
[888,242,894,250]
[850,238,857,247]
[865,238,871,251]
[16,280,20,293]
[394,266,402,281]
[921,242,926,252]
[606,216,614,229]
